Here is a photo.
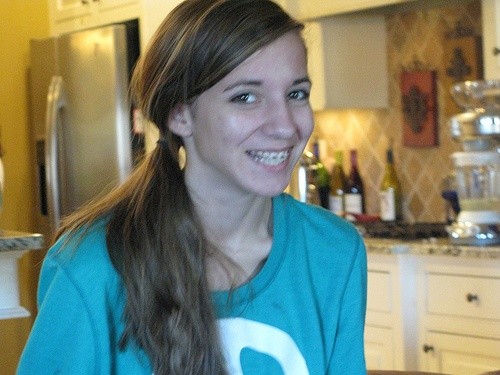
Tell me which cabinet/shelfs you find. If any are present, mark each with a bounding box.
[300,12,388,114]
[362,249,500,375]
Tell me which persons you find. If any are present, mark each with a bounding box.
[16,0,367,375]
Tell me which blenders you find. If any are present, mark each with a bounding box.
[446,79,500,246]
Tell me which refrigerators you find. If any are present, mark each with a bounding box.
[31,19,141,269]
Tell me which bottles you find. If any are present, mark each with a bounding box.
[329,151,344,217]
[378,149,401,222]
[344,151,366,215]
[286,138,329,212]
